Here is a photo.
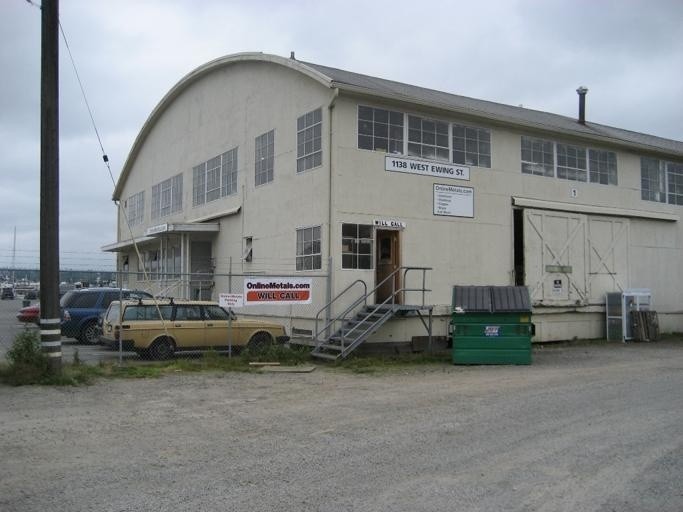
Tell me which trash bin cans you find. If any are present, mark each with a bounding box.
[447,285,535,366]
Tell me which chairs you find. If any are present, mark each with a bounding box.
[181,307,193,318]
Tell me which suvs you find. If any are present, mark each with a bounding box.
[59,288,156,346]
[96,296,291,358]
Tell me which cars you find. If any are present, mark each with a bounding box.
[1,276,116,327]
